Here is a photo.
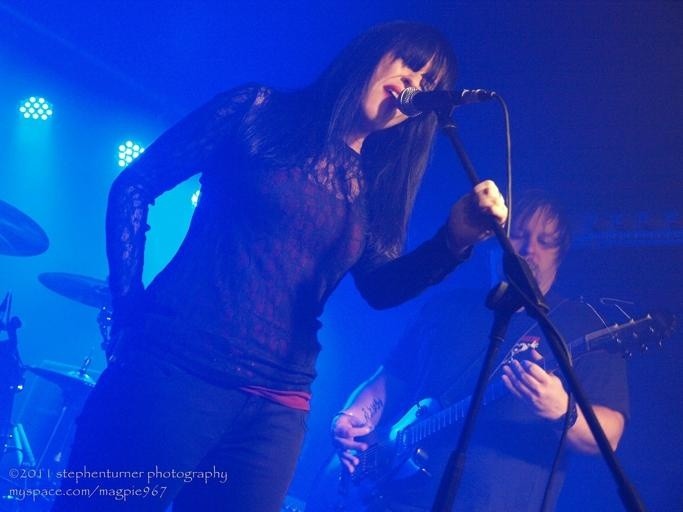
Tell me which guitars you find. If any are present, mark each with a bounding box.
[311,310,676,512]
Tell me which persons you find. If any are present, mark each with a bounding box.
[329,187,631,511]
[46,20,507,511]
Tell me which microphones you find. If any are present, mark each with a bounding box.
[398,84,496,118]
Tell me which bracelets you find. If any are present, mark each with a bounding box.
[334,410,354,416]
[543,392,578,431]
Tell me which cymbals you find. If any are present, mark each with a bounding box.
[1,200,48,255]
[27,365,97,395]
[40,273,115,309]
